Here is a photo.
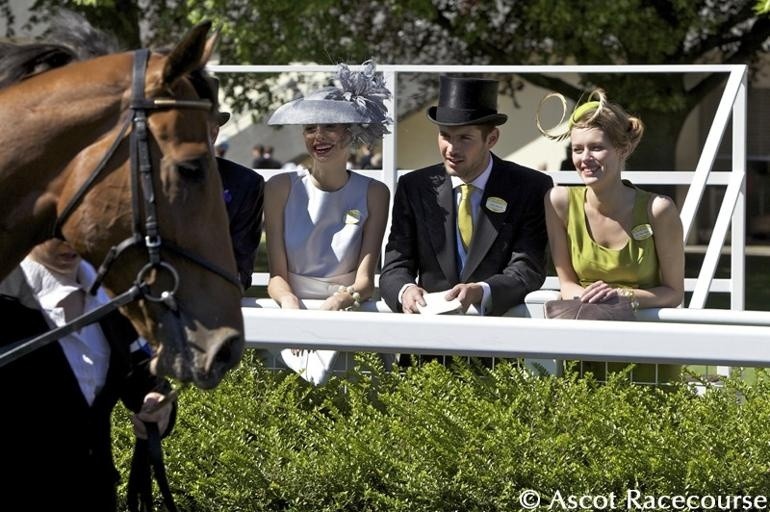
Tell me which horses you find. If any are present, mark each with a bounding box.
[1,18,246,391]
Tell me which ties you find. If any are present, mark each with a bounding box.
[458,185,475,250]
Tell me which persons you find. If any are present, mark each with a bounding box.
[214,143,225,157]
[0,237,178,511]
[250,144,283,169]
[379,122,554,376]
[535,85,684,398]
[190,117,266,294]
[345,142,382,171]
[261,122,396,389]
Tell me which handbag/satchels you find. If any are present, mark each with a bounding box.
[279,347,338,385]
[544,297,636,320]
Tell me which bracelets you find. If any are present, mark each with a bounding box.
[619,286,641,312]
[332,284,361,311]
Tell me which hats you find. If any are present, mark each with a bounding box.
[267,60,391,145]
[426,76,508,127]
[208,77,230,127]
